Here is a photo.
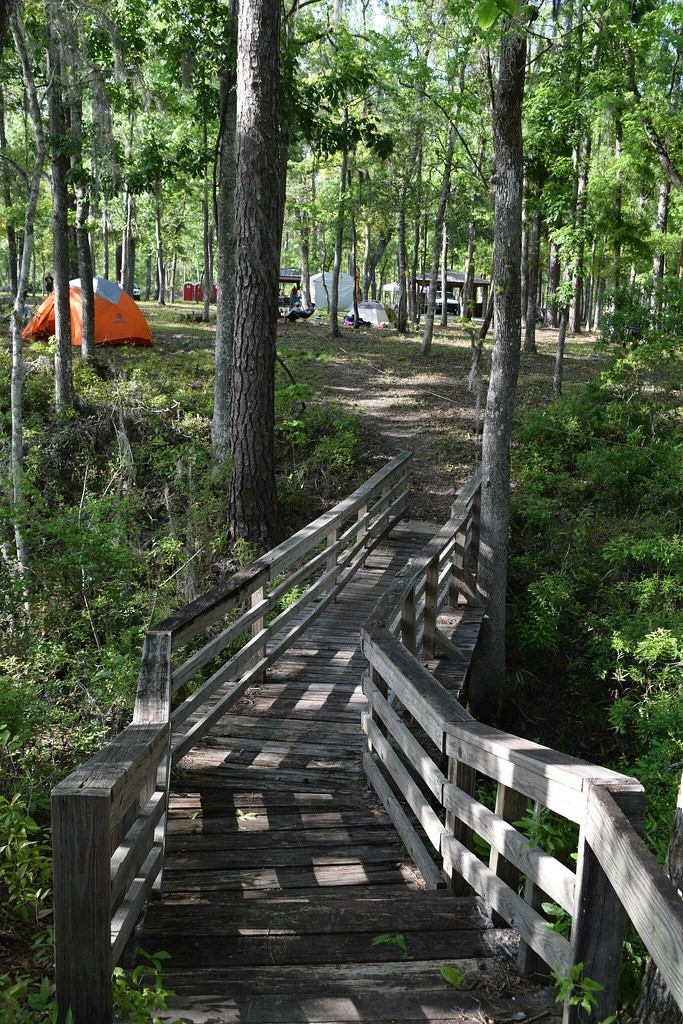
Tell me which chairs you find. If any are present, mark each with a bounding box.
[283,309,315,323]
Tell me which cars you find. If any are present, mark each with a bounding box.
[116,281,142,301]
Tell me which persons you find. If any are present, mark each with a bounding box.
[44,271,54,293]
[292,285,297,296]
[302,303,316,319]
[285,301,302,322]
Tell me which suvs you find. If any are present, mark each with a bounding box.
[420,291,459,316]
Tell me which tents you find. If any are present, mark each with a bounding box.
[343,300,398,329]
[21,276,155,349]
[309,270,355,313]
